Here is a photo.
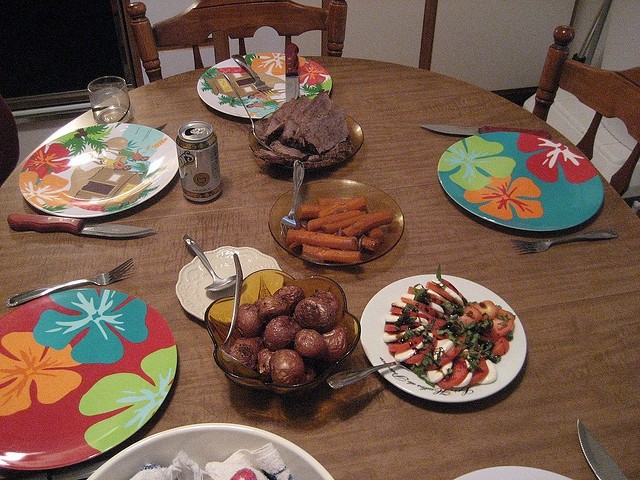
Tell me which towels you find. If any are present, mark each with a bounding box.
[130,444,292,480]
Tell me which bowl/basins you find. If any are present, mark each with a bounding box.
[86,422,335,480]
[264,178,405,268]
[248,111,364,179]
[204,268,361,394]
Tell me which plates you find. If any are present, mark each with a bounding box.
[360,274,528,404]
[447,464,573,480]
[438,132,605,233]
[19,123,179,218]
[197,51,333,120]
[0,287,179,470]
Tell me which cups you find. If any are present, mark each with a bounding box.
[86,76,132,123]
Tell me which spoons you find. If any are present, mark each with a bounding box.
[182,235,236,291]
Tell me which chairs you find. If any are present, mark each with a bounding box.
[532,25,640,196]
[127,0,348,83]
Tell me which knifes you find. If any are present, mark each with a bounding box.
[577,419,629,480]
[285,42,300,106]
[419,124,551,143]
[8,213,156,239]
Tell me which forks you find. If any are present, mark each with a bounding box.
[7,258,134,308]
[512,230,620,256]
[222,73,270,150]
[280,160,305,238]
[322,358,412,391]
[231,53,273,95]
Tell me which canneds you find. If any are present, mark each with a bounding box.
[176,119,224,203]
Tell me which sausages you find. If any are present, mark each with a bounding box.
[284,195,394,264]
